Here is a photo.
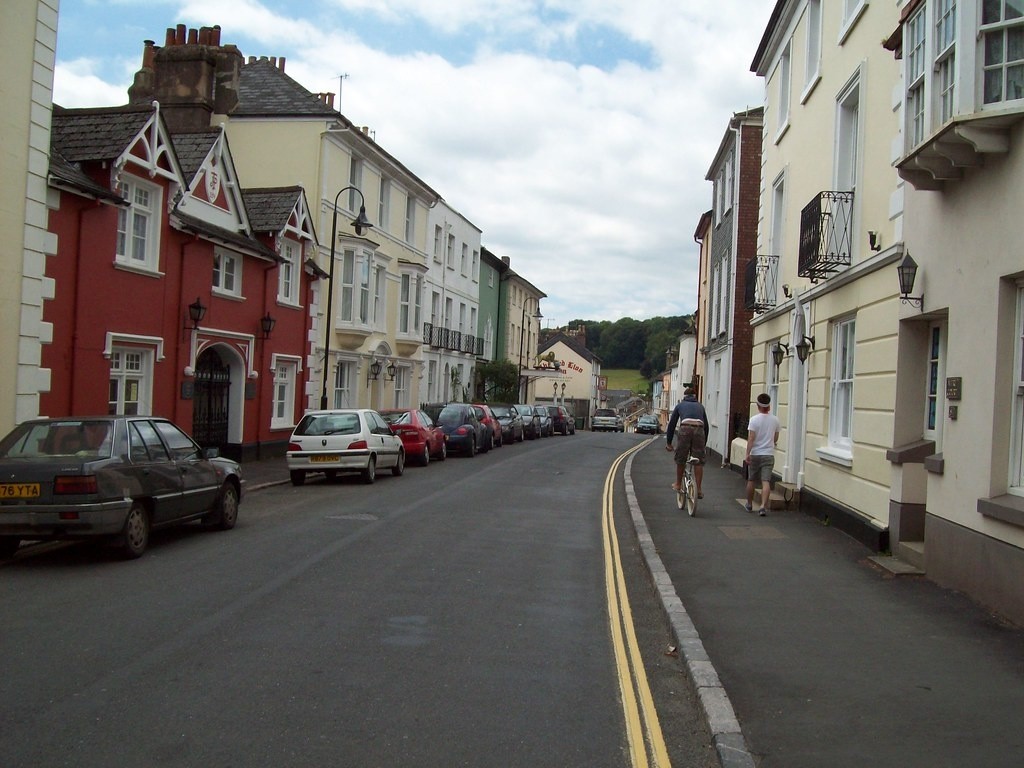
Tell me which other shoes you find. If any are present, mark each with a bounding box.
[745,502,753,512]
[759,508,767,516]
[671,483,680,492]
[698,493,704,500]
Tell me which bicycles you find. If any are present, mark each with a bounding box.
[666,446,702,517]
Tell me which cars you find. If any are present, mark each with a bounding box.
[514,404,542,440]
[636,415,662,434]
[536,407,554,437]
[286,409,405,486]
[424,403,488,457]
[617,415,624,433]
[0,415,247,559]
[489,402,525,445]
[377,409,448,467]
[472,404,503,449]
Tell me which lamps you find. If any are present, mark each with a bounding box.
[869,230,881,252]
[782,284,792,298]
[795,334,815,365]
[367,361,382,388]
[183,297,207,344]
[384,362,398,388]
[897,249,924,312]
[561,383,566,394]
[256,311,276,351]
[553,382,559,395]
[772,341,789,369]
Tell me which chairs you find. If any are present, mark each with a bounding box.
[399,415,408,423]
[382,418,391,425]
[320,422,334,432]
[354,422,368,432]
[59,433,88,456]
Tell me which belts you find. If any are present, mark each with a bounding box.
[681,423,703,428]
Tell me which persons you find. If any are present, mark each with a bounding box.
[666,388,709,498]
[743,394,780,515]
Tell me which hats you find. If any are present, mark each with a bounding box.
[684,389,697,395]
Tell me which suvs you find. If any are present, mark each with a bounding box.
[545,406,575,435]
[591,408,618,432]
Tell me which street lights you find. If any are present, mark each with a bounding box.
[321,186,373,428]
[517,297,544,404]
